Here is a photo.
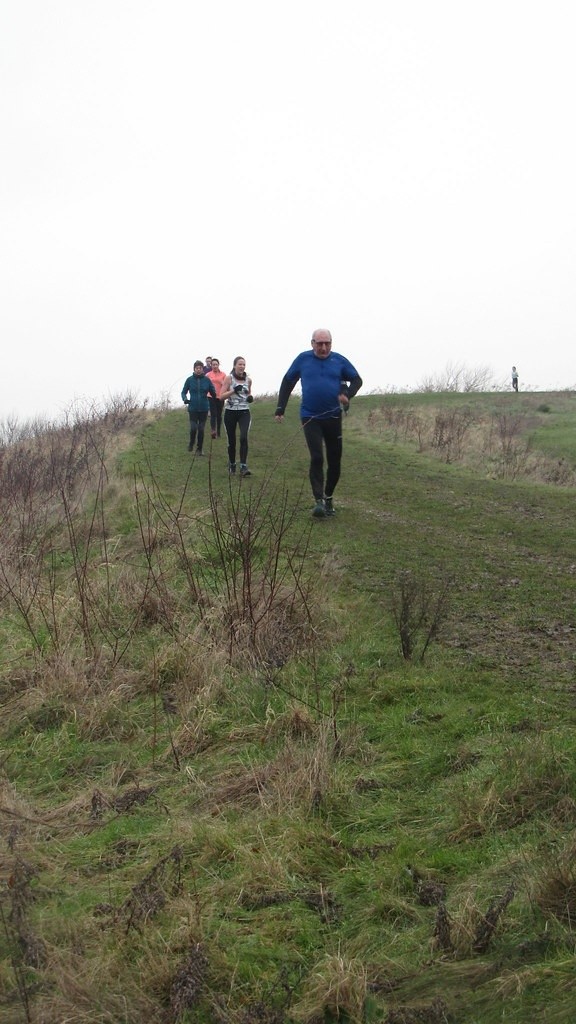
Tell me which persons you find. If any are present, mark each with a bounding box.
[274,329,363,518]
[512,367,519,393]
[204,359,227,439]
[219,356,253,478]
[181,360,216,456]
[203,357,212,374]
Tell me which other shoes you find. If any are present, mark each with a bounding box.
[188,441,194,451]
[312,499,327,518]
[196,448,204,456]
[211,431,217,439]
[325,497,335,515]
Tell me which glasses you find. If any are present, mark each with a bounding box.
[312,340,331,347]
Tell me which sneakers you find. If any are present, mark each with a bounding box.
[239,464,251,478]
[228,462,237,473]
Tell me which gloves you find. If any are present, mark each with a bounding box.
[246,396,253,403]
[184,400,190,404]
[211,393,217,401]
[235,384,243,392]
